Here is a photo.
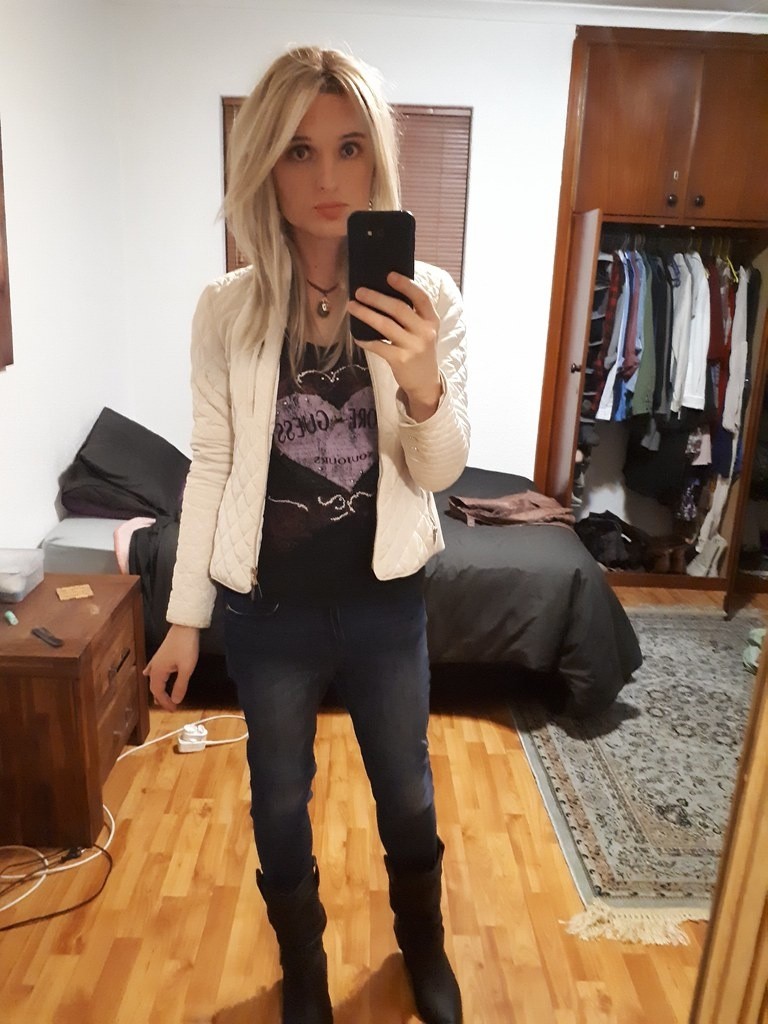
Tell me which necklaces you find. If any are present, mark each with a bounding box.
[307,279,338,317]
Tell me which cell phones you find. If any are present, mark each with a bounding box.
[346,210,416,342]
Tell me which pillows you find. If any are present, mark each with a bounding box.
[61,407,186,519]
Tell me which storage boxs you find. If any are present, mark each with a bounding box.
[0,547,46,601]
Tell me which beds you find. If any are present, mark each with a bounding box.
[44,467,643,723]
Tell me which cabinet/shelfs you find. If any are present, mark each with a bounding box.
[528,26,768,590]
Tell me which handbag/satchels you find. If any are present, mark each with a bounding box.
[448,488,575,528]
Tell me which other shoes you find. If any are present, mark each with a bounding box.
[574,510,728,578]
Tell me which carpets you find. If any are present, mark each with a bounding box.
[515,598,764,946]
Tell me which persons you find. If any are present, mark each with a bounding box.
[142,46,471,1024]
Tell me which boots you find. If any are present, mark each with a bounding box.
[256,856,333,1024]
[383,834,463,1024]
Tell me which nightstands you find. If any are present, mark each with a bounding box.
[1,571,147,852]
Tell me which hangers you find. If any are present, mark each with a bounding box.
[603,225,740,285]
